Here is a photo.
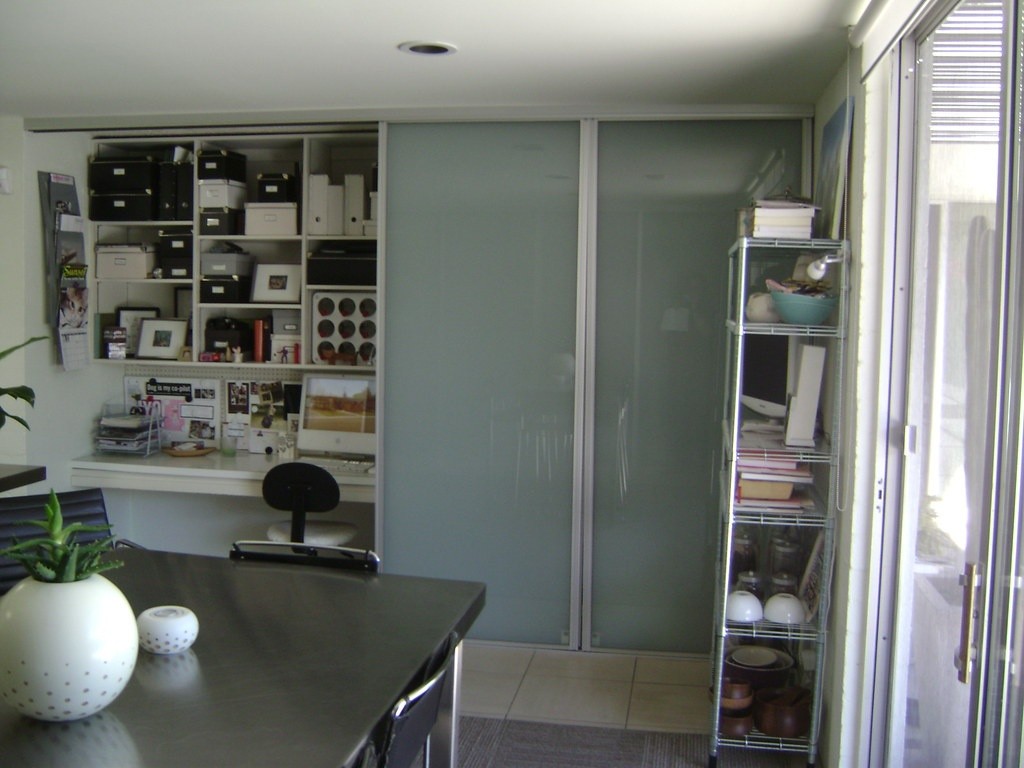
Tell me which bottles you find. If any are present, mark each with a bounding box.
[733,531,801,575]
[737,570,764,600]
[770,572,797,598]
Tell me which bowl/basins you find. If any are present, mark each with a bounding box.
[768,292,833,325]
[706,644,811,737]
[726,589,762,622]
[763,593,805,623]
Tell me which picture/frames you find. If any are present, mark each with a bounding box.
[117,307,160,358]
[249,262,302,305]
[135,317,190,361]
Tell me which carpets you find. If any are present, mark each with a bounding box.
[412,714,824,768]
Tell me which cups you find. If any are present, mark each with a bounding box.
[220,437,237,456]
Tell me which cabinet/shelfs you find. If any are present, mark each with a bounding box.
[95,400,163,456]
[708,236,851,768]
[82,133,386,371]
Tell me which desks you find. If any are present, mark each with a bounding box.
[0,463,47,492]
[72,449,376,503]
[0,548,490,768]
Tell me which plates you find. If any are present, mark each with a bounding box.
[162,447,216,457]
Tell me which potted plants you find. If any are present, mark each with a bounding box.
[0,488,138,722]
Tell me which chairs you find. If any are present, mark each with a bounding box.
[0,487,148,596]
[352,632,458,768]
[262,463,359,547]
[228,541,381,574]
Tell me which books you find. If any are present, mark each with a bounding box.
[38,171,90,371]
[732,454,815,514]
[95,415,161,450]
[749,200,822,240]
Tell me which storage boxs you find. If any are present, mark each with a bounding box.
[88,148,376,303]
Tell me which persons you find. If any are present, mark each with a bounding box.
[277,347,288,364]
